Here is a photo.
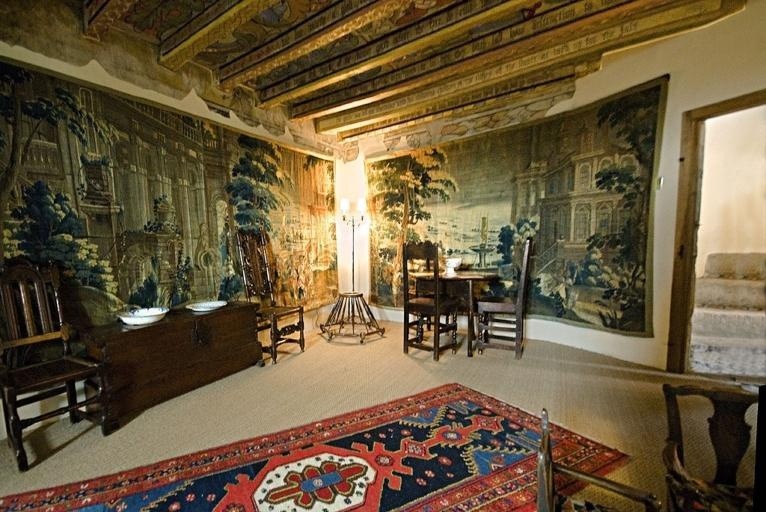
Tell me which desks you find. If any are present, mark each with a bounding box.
[412,269,496,358]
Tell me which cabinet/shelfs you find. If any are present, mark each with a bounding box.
[77,299,262,436]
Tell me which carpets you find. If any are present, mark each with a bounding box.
[0,383,630,509]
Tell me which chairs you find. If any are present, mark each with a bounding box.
[401,240,458,364]
[1,256,112,474]
[534,379,765,510]
[236,226,304,365]
[474,237,534,360]
